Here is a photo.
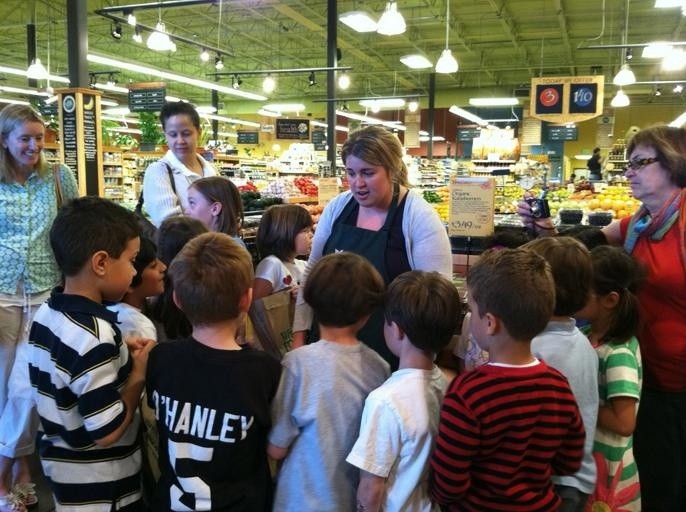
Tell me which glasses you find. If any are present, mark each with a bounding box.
[626,157,658,171]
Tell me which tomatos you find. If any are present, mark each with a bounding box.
[294,177,319,197]
[238,182,256,192]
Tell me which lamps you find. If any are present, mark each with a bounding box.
[339,10,377,33]
[435,2,459,74]
[25,12,47,80]
[1,67,260,139]
[399,54,433,69]
[148,7,175,53]
[216,56,224,69]
[201,48,208,62]
[610,1,640,86]
[111,22,121,40]
[128,9,135,27]
[133,26,142,43]
[377,2,406,36]
[610,27,634,110]
[267,67,523,155]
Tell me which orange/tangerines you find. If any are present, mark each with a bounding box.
[585,197,637,220]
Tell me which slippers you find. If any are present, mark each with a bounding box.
[0,482,39,511]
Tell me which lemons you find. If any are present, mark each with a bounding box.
[434,205,449,222]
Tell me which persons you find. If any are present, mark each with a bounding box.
[187,176,244,247]
[516,127,686,512]
[292,123,452,371]
[147,101,216,228]
[30,198,585,511]
[2,102,80,512]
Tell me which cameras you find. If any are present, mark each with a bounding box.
[525,198,551,219]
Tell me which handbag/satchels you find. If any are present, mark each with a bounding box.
[133,209,158,247]
[250,287,296,359]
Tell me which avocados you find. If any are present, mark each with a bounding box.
[240,191,282,213]
